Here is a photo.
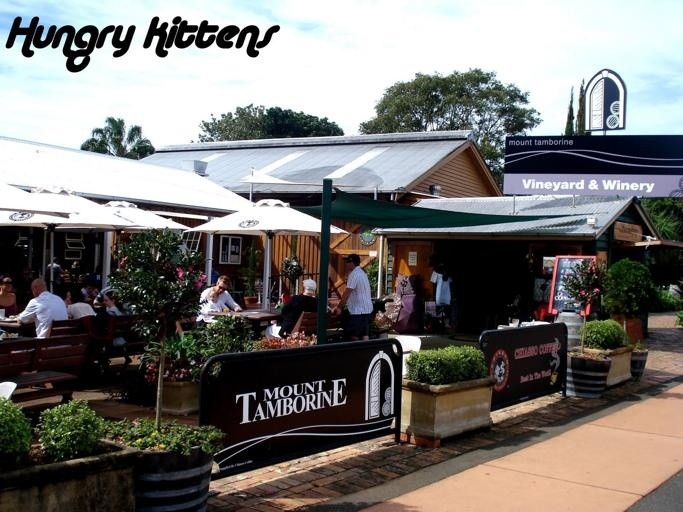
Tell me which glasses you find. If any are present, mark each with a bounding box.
[219,284,227,291]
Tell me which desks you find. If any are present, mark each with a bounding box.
[204,305,279,340]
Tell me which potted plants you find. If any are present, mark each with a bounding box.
[400,344,496,446]
[0,226,251,511]
[575,257,651,387]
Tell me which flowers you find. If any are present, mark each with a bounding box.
[559,257,606,355]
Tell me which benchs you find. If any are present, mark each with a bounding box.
[289,311,343,345]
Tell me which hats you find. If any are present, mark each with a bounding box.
[343,254,360,265]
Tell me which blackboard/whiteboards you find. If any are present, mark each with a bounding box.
[548,255,596,316]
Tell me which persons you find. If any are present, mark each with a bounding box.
[265,279,318,339]
[0,275,127,337]
[195,275,243,328]
[335,254,373,340]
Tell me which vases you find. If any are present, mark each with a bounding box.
[562,353,610,401]
